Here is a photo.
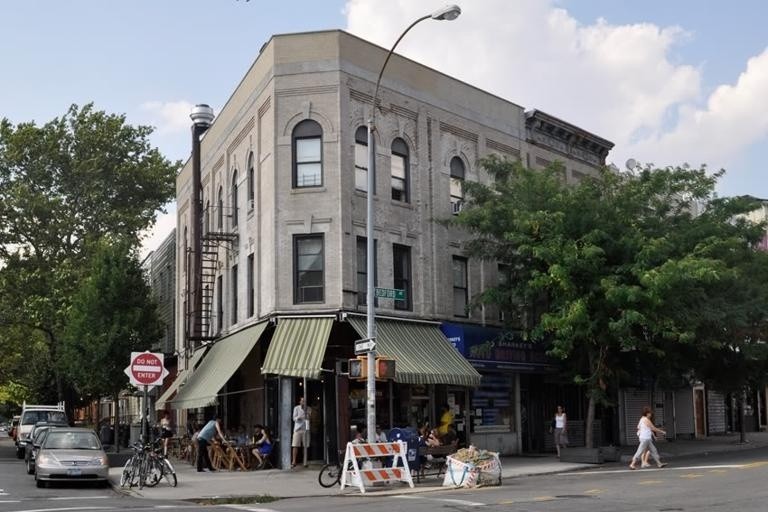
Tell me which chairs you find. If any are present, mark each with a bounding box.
[162,438,277,471]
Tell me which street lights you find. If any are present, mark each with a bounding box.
[185,103,215,353]
[363,5,466,486]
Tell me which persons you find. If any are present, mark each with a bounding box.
[290,397,321,468]
[159,412,173,458]
[637,429,657,468]
[349,403,457,447]
[549,404,567,457]
[628,406,667,470]
[187,412,274,472]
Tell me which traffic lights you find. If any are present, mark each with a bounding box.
[376,357,396,379]
[348,358,368,379]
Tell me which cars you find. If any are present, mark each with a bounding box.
[0,400,70,475]
[35,427,108,488]
[92,413,175,446]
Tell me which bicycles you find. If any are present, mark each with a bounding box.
[119,435,179,492]
[318,446,362,488]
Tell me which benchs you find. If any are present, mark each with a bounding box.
[410,443,455,484]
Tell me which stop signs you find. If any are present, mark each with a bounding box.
[124,349,170,389]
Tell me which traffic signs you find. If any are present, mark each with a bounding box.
[355,337,376,355]
[374,286,406,302]
